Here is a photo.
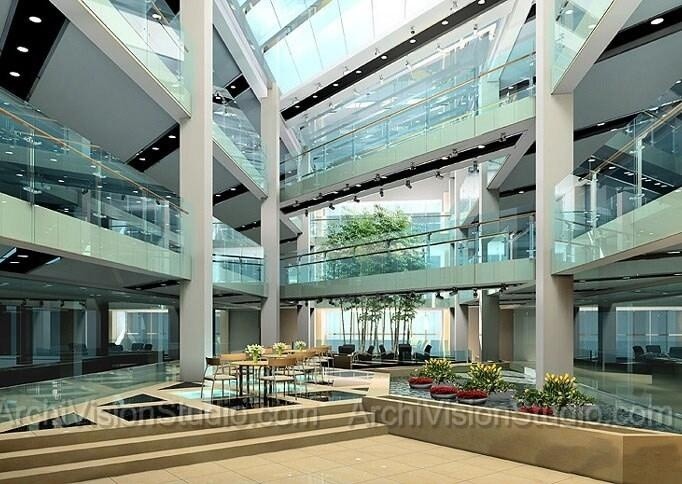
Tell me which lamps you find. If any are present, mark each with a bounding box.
[293,173,384,217]
[304,298,338,307]
[433,285,508,300]
[404,148,481,190]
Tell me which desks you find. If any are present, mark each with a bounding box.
[399,343,411,361]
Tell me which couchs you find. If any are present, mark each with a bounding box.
[332,352,354,368]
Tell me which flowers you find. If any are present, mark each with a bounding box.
[293,340,306,351]
[272,342,287,356]
[520,370,595,416]
[408,357,518,398]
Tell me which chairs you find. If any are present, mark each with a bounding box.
[69,342,88,353]
[632,345,682,375]
[413,344,432,360]
[200,344,334,405]
[339,344,394,361]
[109,342,153,352]
